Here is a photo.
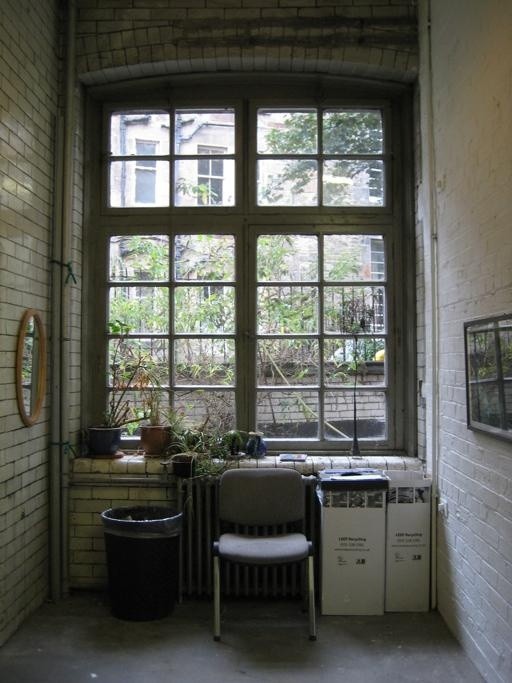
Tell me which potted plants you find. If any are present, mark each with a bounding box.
[221,429,246,460]
[162,409,224,479]
[87,319,160,453]
[137,361,171,455]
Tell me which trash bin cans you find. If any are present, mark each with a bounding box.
[100,505,184,622]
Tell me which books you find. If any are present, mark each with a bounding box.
[280,454,307,461]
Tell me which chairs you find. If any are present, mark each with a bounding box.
[212,467,316,643]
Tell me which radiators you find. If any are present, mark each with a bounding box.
[176,474,318,605]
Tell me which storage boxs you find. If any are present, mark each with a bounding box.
[383,470,431,612]
[317,468,387,617]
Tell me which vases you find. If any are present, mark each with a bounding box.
[247,431,267,459]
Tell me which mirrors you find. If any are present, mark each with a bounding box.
[16,308,47,427]
[463,313,512,443]
